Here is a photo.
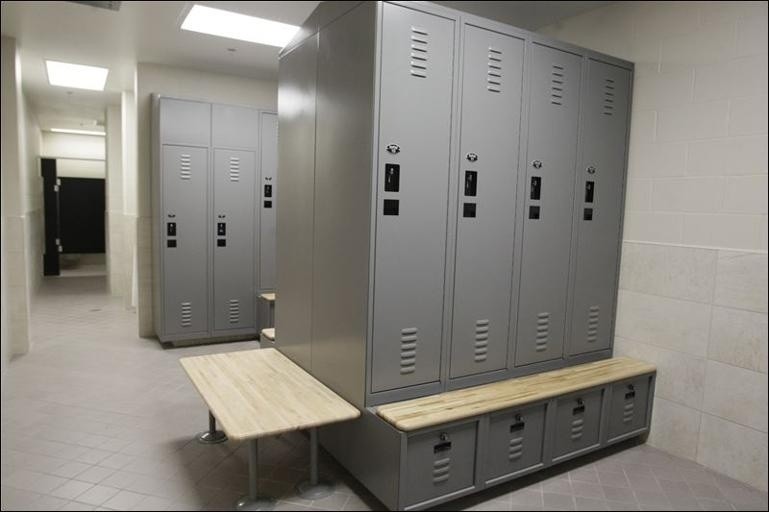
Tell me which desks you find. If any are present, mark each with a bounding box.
[177,346,360,507]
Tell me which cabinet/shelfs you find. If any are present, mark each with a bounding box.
[393,373,654,500]
[370,9,627,405]
[155,100,280,348]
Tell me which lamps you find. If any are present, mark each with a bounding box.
[178,5,297,54]
